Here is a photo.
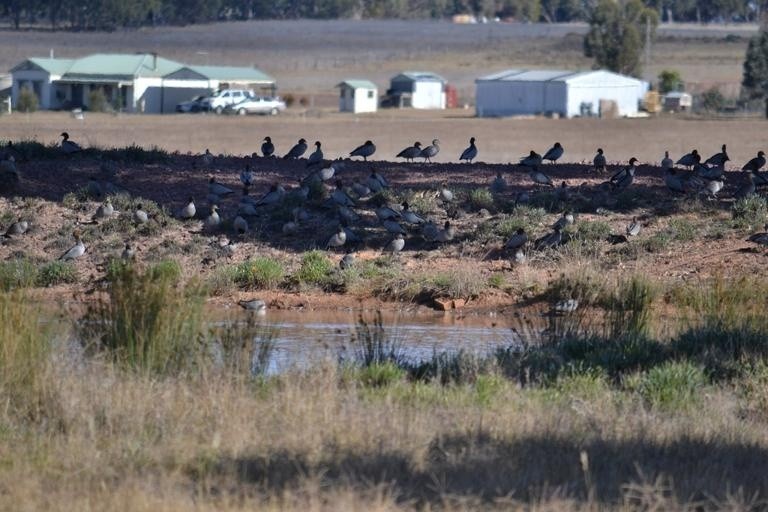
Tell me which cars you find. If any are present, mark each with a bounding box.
[176,89,288,116]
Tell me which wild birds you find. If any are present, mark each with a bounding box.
[209,169,456,256]
[122,242,137,263]
[172,196,198,218]
[548,290,581,319]
[91,199,114,222]
[419,138,440,164]
[0,220,29,239]
[488,141,767,211]
[460,137,478,163]
[397,140,422,163]
[349,140,376,160]
[133,203,150,227]
[60,131,83,161]
[261,135,276,158]
[58,234,86,264]
[0,140,19,177]
[200,205,224,233]
[309,141,324,163]
[287,137,308,160]
[239,297,268,318]
[500,209,576,253]
